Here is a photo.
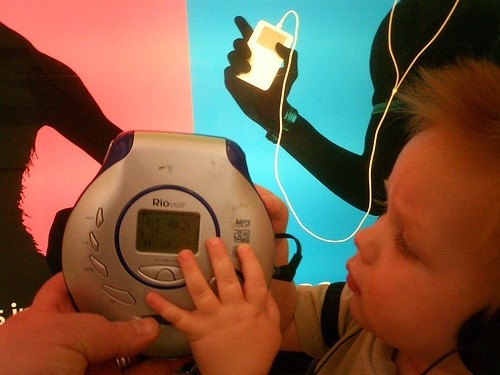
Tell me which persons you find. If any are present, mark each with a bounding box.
[0,266,188,373]
[2,19,129,324]
[225,0,499,219]
[144,58,499,375]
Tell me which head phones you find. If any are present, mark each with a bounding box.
[459,310,500,375]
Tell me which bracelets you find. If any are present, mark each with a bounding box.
[265,104,299,142]
